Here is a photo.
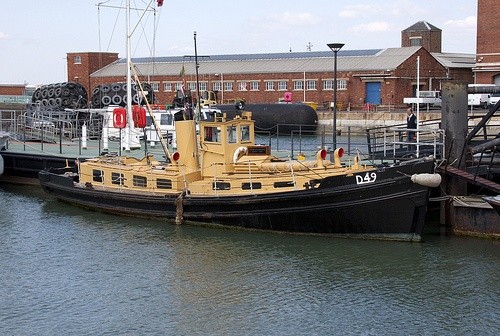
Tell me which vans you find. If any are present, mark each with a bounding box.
[487,86,500,110]
[466,84,497,110]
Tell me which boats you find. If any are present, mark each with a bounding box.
[45,29,442,243]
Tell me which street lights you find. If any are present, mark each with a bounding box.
[326,42,345,151]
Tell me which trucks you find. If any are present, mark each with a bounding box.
[403,90,442,110]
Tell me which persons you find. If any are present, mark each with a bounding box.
[405,108,417,152]
